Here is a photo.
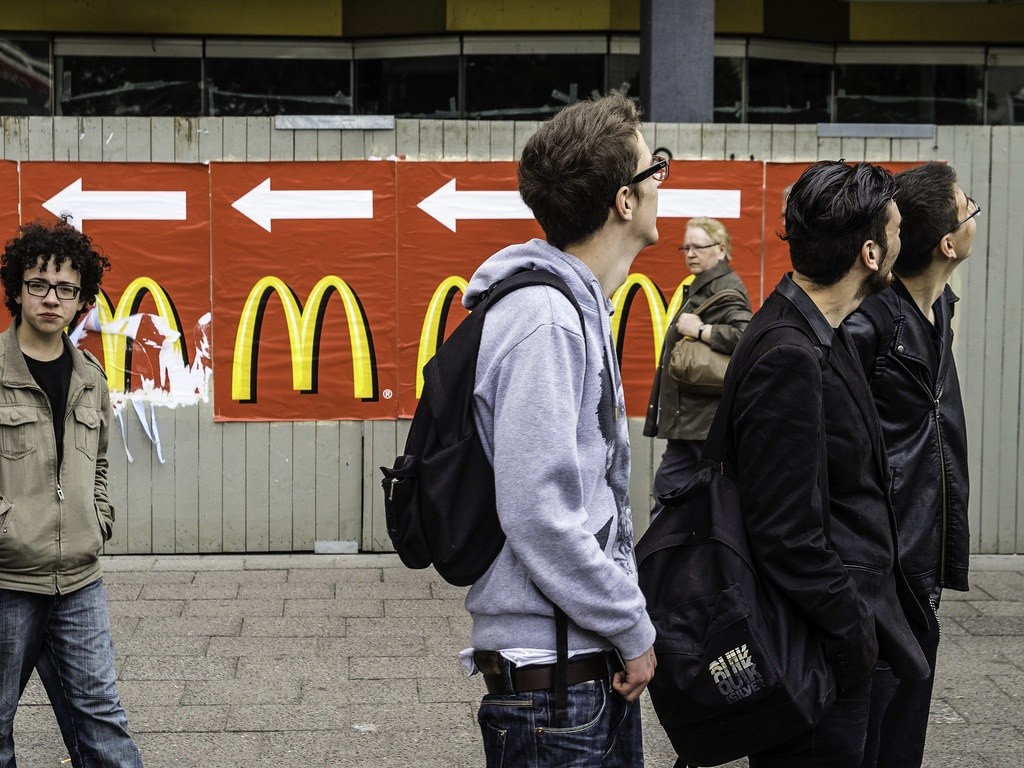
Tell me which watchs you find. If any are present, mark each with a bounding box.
[697,323,706,341]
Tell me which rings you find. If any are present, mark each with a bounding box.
[675,323,679,327]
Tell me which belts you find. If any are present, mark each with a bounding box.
[481,647,623,696]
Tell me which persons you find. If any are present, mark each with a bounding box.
[693,162,940,768]
[0,213,145,768]
[841,161,981,768]
[458,86,658,768]
[641,214,755,527]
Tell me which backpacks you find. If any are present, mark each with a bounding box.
[380,269,585,588]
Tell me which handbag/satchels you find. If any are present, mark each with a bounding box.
[633,320,833,768]
[668,288,750,396]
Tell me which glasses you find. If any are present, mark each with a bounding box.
[933,196,982,254]
[678,241,720,256]
[609,154,670,209]
[20,276,84,300]
